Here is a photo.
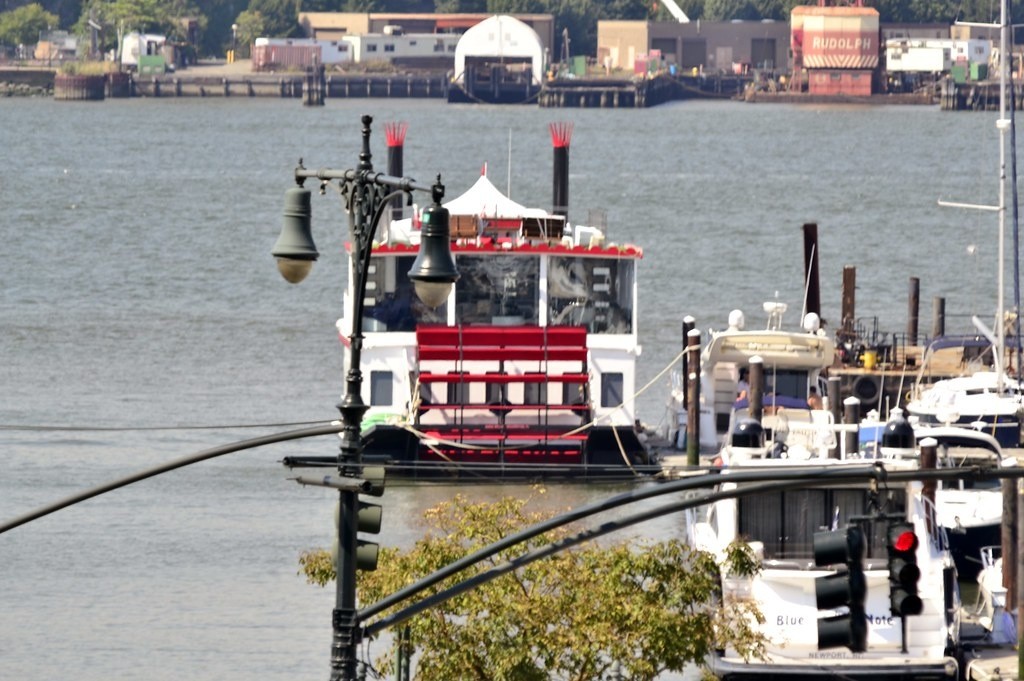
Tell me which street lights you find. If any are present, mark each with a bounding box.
[269,113,461,680]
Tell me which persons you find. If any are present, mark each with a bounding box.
[734,370,784,416]
[779,74,786,91]
[635,418,646,433]
[665,59,699,83]
[604,53,612,74]
[833,326,862,348]
[807,386,823,410]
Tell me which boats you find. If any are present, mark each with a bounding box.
[662,427,964,681]
[940,77,1024,114]
[665,299,1020,569]
[332,174,649,475]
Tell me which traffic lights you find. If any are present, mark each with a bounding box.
[889,518,924,617]
[814,525,872,656]
